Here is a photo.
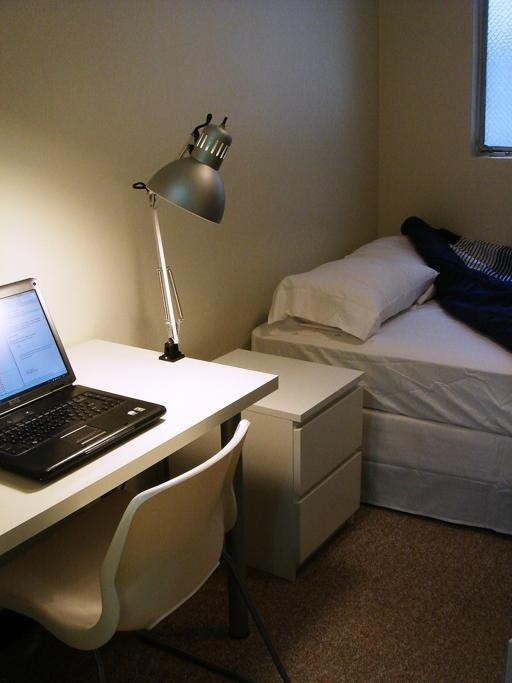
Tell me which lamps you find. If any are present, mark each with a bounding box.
[133,112,234,364]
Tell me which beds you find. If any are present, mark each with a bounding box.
[250,217,512,540]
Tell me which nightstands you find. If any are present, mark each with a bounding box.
[205,347,367,582]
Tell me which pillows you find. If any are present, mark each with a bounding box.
[267,234,442,342]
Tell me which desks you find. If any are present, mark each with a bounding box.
[0,338,280,683]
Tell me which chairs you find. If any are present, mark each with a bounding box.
[0,418,293,683]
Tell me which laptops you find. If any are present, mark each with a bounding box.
[0,278,166,480]
